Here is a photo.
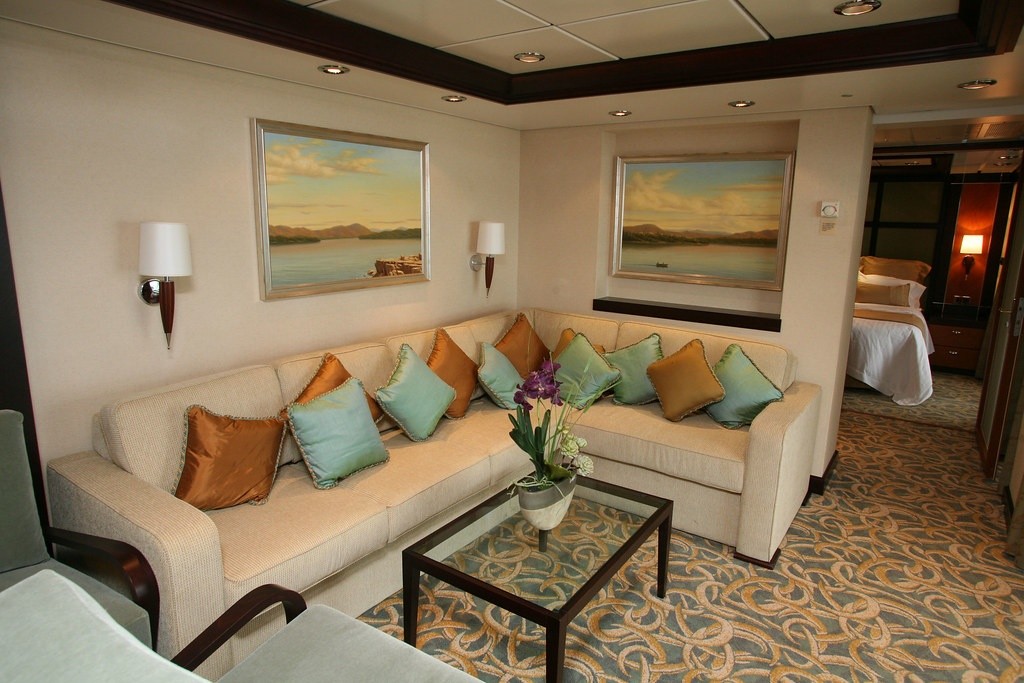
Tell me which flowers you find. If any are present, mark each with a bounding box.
[508,362,594,485]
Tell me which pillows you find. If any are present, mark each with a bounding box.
[171,404,287,513]
[859,255,931,282]
[474,343,526,410]
[857,270,927,308]
[855,282,911,307]
[278,351,391,491]
[425,327,479,421]
[646,338,725,423]
[494,311,549,379]
[600,334,664,406]
[705,344,785,430]
[374,342,458,443]
[550,327,623,410]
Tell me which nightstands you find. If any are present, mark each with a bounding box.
[928,318,986,376]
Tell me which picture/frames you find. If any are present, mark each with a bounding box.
[250,117,433,301]
[608,151,795,292]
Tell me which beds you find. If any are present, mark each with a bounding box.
[845,256,936,406]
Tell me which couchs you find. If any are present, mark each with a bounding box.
[42,308,822,681]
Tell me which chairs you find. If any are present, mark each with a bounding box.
[0,568,484,683]
[0,410,160,654]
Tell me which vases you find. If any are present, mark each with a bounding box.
[516,464,578,530]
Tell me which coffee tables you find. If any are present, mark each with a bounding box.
[399,472,673,683]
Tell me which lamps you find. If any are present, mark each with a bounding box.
[137,222,193,351]
[960,234,983,281]
[469,220,505,299]
